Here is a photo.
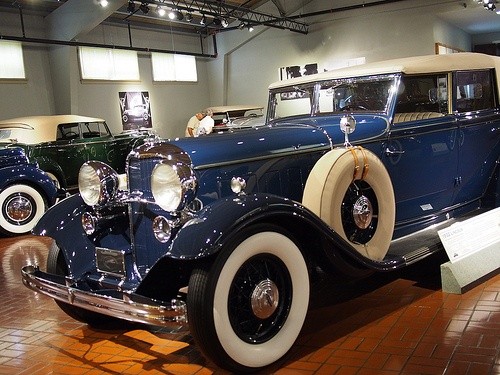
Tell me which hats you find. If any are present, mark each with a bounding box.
[200,111,207,117]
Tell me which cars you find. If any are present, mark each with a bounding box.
[21,51,500,371]
[204,105,270,136]
[0,114,160,235]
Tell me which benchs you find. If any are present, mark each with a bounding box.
[392,113,445,123]
[216,124,225,126]
[394,96,492,112]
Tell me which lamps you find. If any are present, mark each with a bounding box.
[97,0,254,32]
[470,0,500,15]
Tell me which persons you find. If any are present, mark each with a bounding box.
[200,108,214,135]
[185,107,207,138]
